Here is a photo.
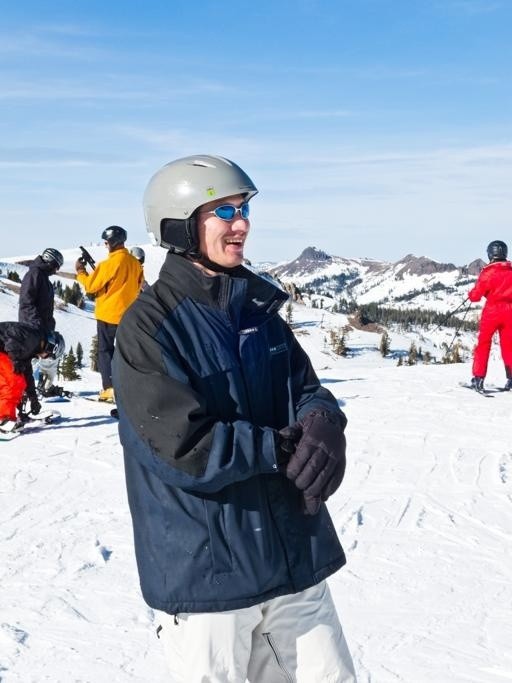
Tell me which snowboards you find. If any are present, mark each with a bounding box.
[45,397,69,403]
[0,409,61,440]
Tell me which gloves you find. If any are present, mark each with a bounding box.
[277,409,350,516]
[76,258,87,271]
[11,359,29,374]
[30,400,41,414]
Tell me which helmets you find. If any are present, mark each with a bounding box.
[42,247,62,269]
[131,247,146,262]
[102,226,128,248]
[145,157,260,260]
[43,332,67,359]
[486,241,508,260]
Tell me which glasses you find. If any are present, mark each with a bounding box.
[197,201,252,222]
[105,241,109,245]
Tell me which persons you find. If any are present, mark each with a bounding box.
[468,238,511,394]
[129,246,151,294]
[1,321,65,441]
[17,247,69,393]
[109,152,356,680]
[75,226,144,402]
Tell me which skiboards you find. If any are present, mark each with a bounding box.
[459,379,512,397]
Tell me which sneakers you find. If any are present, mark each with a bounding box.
[0,418,23,434]
[472,376,485,392]
[99,387,116,403]
[505,379,512,390]
